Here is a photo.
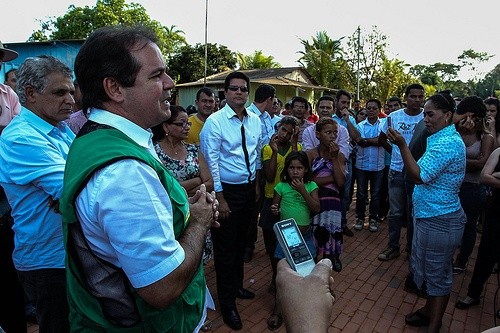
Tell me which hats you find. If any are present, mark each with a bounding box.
[0,41,19,63]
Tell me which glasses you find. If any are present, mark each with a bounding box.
[273,102,279,105]
[367,106,377,110]
[294,106,304,109]
[227,86,248,92]
[168,122,192,127]
[360,112,368,117]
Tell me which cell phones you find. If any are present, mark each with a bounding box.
[387,116,392,128]
[274,217,314,277]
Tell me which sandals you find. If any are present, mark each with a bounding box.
[405,306,433,327]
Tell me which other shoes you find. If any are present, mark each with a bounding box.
[403,271,428,298]
[453,265,467,276]
[368,218,379,232]
[323,253,343,274]
[376,214,386,222]
[244,251,254,263]
[455,297,481,310]
[269,281,277,297]
[267,312,283,331]
[343,226,354,238]
[355,219,366,231]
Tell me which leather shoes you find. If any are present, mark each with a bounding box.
[238,288,255,301]
[221,310,243,330]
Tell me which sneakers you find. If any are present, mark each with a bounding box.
[377,246,401,261]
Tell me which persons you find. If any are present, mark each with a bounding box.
[386,93,466,333]
[262,116,304,294]
[332,82,456,300]
[246,83,320,213]
[0,41,43,333]
[62,77,92,136]
[152,86,227,262]
[268,150,321,329]
[0,56,76,333]
[452,94,500,308]
[303,96,351,161]
[275,257,335,333]
[63,27,221,333]
[200,71,262,331]
[305,118,348,272]
